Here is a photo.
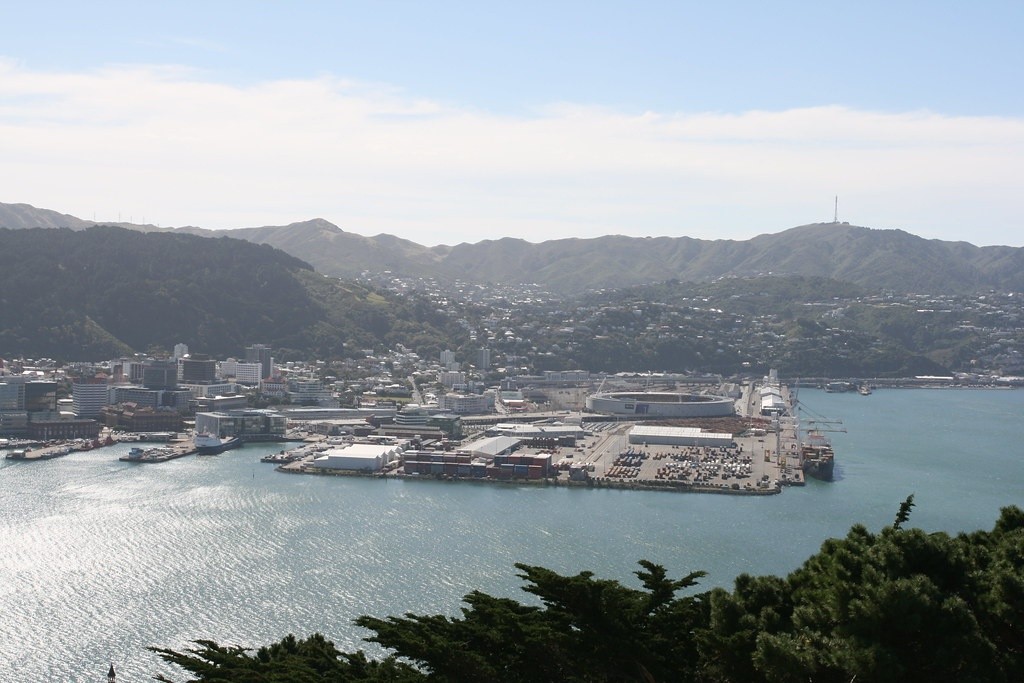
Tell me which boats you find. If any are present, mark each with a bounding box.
[798,421,835,482]
